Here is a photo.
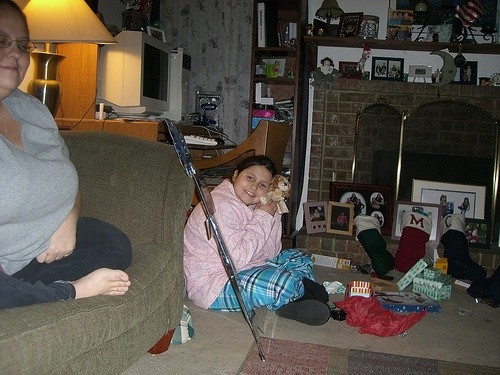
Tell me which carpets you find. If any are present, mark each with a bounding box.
[236,337,500,375]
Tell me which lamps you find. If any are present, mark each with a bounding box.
[22,0,120,118]
[316,0,344,33]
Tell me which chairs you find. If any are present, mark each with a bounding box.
[191,119,293,204]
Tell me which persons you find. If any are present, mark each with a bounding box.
[314,209,322,221]
[183,155,331,327]
[343,18,376,37]
[0,0,133,310]
[337,211,349,226]
[376,63,399,77]
[310,57,338,90]
[347,193,383,225]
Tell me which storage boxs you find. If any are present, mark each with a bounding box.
[255,82,274,105]
[311,240,473,312]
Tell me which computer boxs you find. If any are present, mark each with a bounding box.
[160,49,191,122]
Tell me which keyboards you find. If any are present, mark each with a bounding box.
[183,135,218,146]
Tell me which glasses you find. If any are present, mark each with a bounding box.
[0,35,37,53]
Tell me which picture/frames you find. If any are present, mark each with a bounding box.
[327,201,353,236]
[411,179,487,220]
[337,12,363,38]
[372,57,404,81]
[464,218,492,249]
[391,201,442,245]
[389,0,500,36]
[452,61,477,85]
[146,26,166,43]
[303,201,327,234]
[329,181,393,236]
[339,61,361,79]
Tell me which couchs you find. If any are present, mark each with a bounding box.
[0,131,195,375]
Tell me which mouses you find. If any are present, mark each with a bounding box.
[214,138,224,145]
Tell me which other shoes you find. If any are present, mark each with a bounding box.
[302,278,328,302]
[276,299,331,325]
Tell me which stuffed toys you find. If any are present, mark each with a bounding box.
[260,175,291,215]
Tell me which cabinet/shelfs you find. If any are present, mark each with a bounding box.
[248,0,309,239]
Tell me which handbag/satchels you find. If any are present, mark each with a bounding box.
[335,280,425,337]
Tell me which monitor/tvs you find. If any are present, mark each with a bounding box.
[94,30,171,120]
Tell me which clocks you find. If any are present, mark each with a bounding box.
[490,73,500,86]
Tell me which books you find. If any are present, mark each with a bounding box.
[275,99,294,119]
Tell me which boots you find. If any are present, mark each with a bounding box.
[354,215,395,277]
[440,214,486,280]
[394,212,432,273]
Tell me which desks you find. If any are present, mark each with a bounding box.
[55,118,237,162]
